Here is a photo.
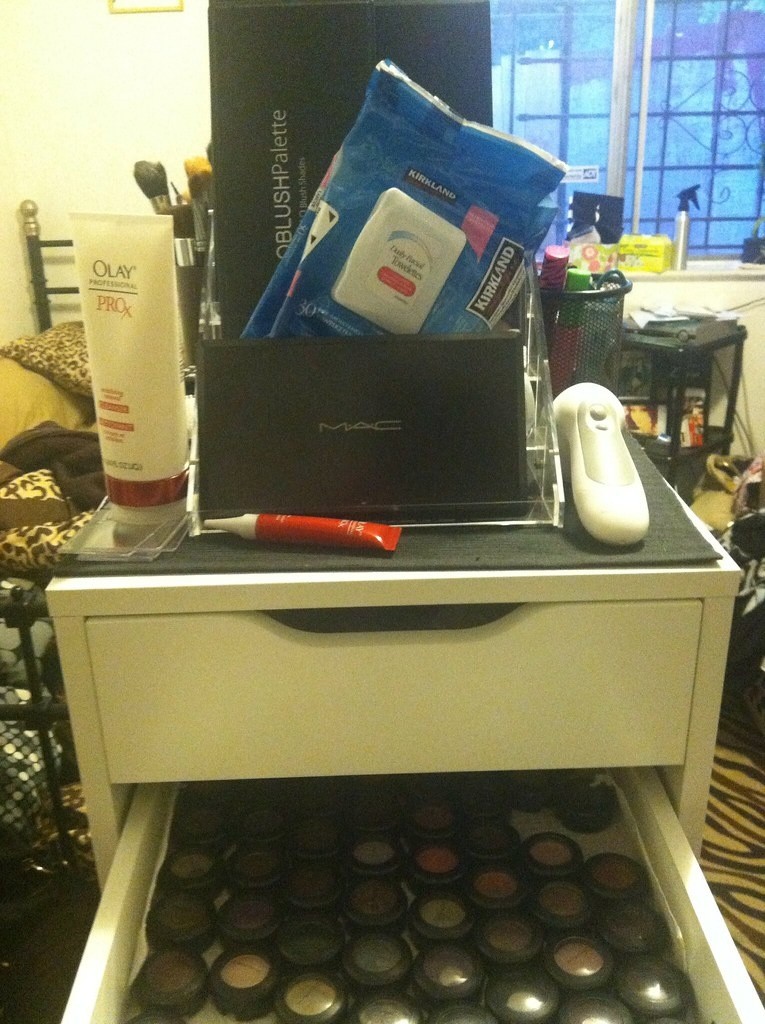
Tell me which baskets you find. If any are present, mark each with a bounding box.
[540,269,630,390]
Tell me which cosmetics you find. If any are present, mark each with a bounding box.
[128,773,691,1024]
[68,212,191,526]
[205,515,400,554]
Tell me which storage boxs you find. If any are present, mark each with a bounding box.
[196,332,527,522]
[618,233,672,273]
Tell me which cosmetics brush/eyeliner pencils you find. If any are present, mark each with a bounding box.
[135,144,211,361]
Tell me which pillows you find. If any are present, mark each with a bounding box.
[0,468,95,576]
[0,319,94,399]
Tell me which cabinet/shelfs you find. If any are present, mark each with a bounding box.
[619,326,747,486]
[45,418,765,1024]
